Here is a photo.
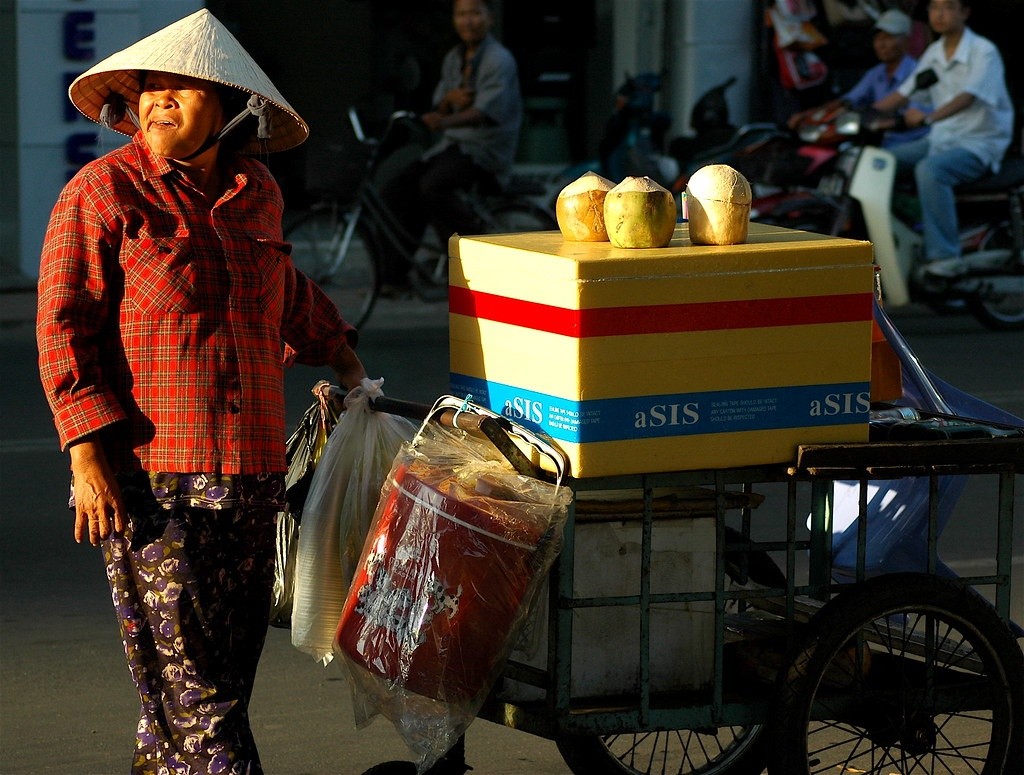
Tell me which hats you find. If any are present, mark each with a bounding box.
[876,9,912,36]
[68,8,310,156]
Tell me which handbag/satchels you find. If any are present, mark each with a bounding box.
[290,379,422,661]
[268,383,347,629]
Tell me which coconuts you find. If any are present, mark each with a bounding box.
[556,170,618,242]
[603,176,676,248]
[685,165,753,245]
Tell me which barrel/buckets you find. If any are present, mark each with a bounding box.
[334,395,569,704]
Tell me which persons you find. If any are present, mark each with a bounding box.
[373,0,522,300]
[36,9,367,775]
[788,0,1014,278]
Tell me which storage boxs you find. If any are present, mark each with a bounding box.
[871,265,886,341]
[446,220,875,481]
[872,341,902,401]
[501,486,716,705]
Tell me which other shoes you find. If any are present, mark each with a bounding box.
[918,258,967,278]
